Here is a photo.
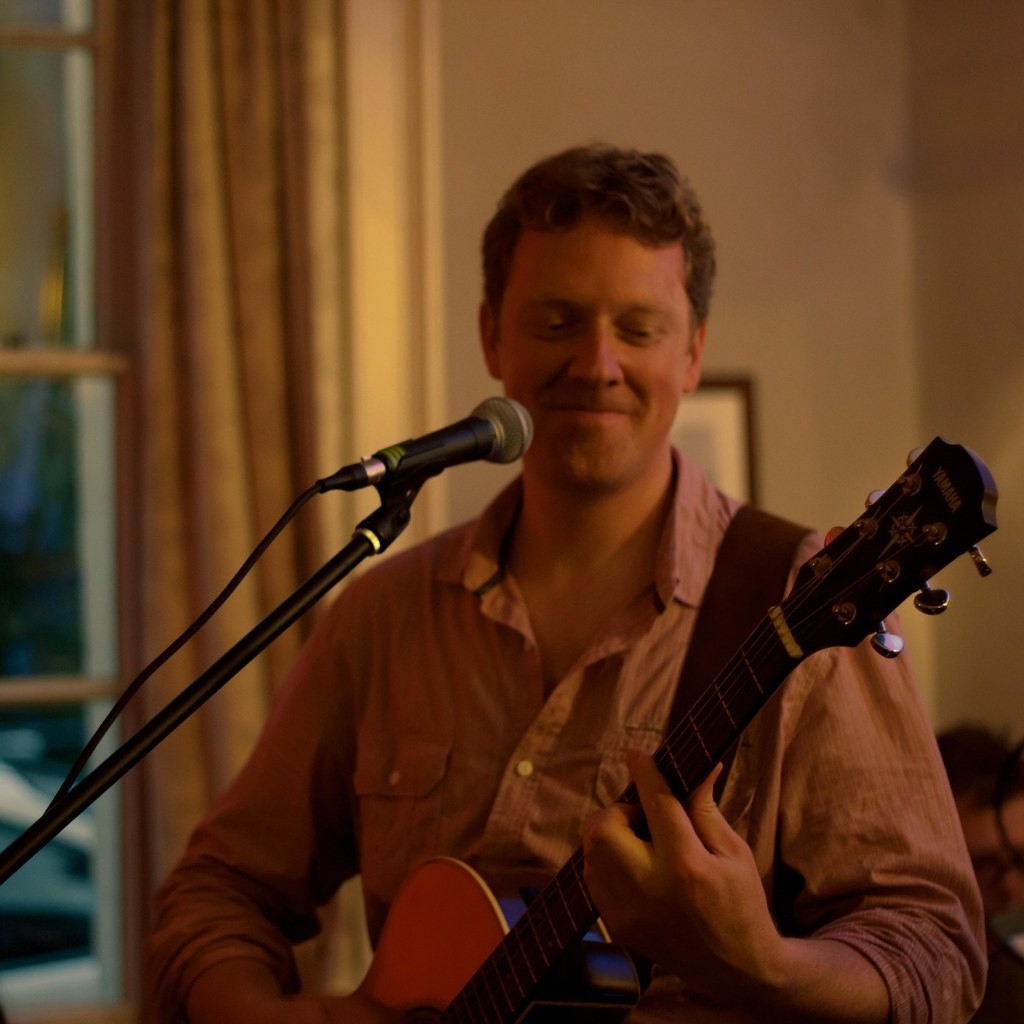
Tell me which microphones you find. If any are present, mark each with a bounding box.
[316,397,533,493]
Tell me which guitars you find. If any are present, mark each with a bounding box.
[352,425,1001,1024]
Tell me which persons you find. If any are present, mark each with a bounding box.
[153,141,987,1023]
